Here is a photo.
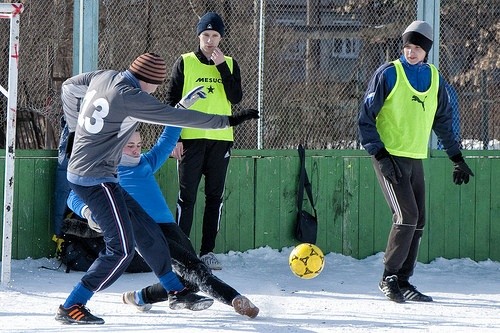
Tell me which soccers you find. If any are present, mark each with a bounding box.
[288,242,325,279]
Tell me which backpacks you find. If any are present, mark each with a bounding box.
[53,205,107,274]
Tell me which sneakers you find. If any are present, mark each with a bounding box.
[168,287,214,311]
[378,274,405,303]
[123,292,152,311]
[232,296,260,319]
[55,304,105,323]
[399,280,433,302]
[199,252,222,270]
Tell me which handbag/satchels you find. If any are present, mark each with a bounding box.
[293,210,318,245]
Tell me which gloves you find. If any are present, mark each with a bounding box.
[83,207,103,234]
[374,148,402,185]
[175,86,206,110]
[65,131,76,159]
[228,108,259,126]
[449,152,474,185]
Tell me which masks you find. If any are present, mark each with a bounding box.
[120,153,141,168]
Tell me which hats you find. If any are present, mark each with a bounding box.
[401,21,434,55]
[129,52,167,86]
[196,11,224,37]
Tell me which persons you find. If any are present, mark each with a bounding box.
[358,20,473,304]
[166,12,242,270]
[55,53,260,326]
[67,84,259,320]
